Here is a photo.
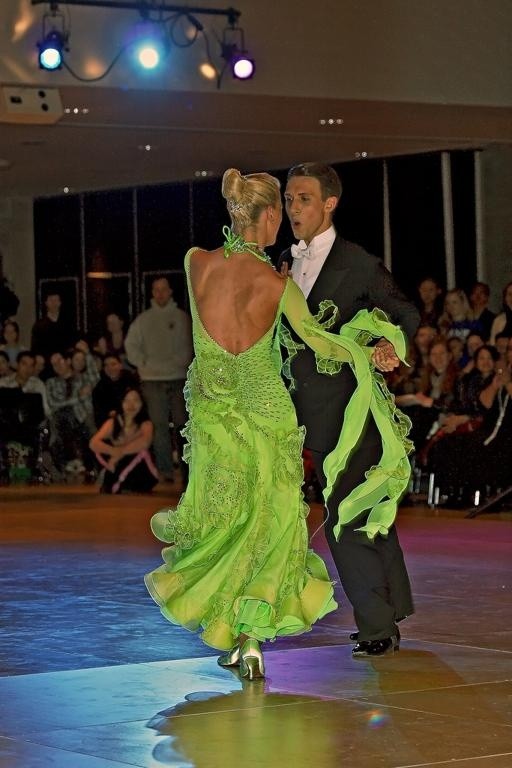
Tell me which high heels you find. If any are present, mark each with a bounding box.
[238,639,264,682]
[217,645,238,668]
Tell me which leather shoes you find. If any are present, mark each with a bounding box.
[349,612,414,658]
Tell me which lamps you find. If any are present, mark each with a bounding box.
[30,1,255,90]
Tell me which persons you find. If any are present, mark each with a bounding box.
[144,167,417,684]
[273,161,422,655]
[296,279,512,515]
[1,277,192,498]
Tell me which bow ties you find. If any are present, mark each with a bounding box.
[289,245,324,259]
